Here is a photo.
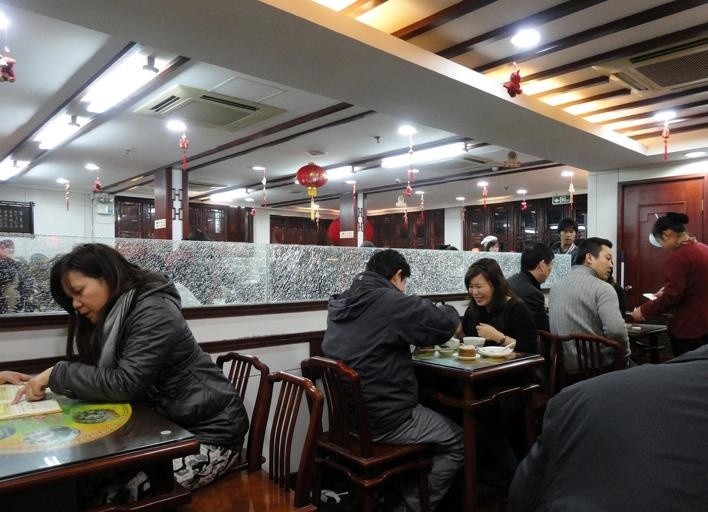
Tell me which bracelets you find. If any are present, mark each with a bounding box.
[498,335,507,346]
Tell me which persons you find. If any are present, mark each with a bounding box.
[547,236,640,380]
[629,211,708,352]
[12,243,249,505]
[1,371,29,385]
[507,345,708,510]
[606,261,628,323]
[320,249,467,512]
[457,258,537,352]
[0,217,579,313]
[505,243,554,334]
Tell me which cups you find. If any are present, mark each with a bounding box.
[457,345,476,356]
[446,337,459,350]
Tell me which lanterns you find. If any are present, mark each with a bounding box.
[296,164,329,222]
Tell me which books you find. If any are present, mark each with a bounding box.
[0,382,64,421]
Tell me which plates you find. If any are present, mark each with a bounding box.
[420,349,437,353]
[476,346,512,359]
[70,409,119,426]
[0,426,17,441]
[452,352,481,361]
[20,426,80,449]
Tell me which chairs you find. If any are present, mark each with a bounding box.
[518,330,557,445]
[175,372,325,511]
[215,353,270,472]
[301,356,433,511]
[558,333,627,378]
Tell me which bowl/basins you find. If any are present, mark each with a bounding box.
[438,348,452,357]
[462,336,485,347]
[409,345,416,354]
